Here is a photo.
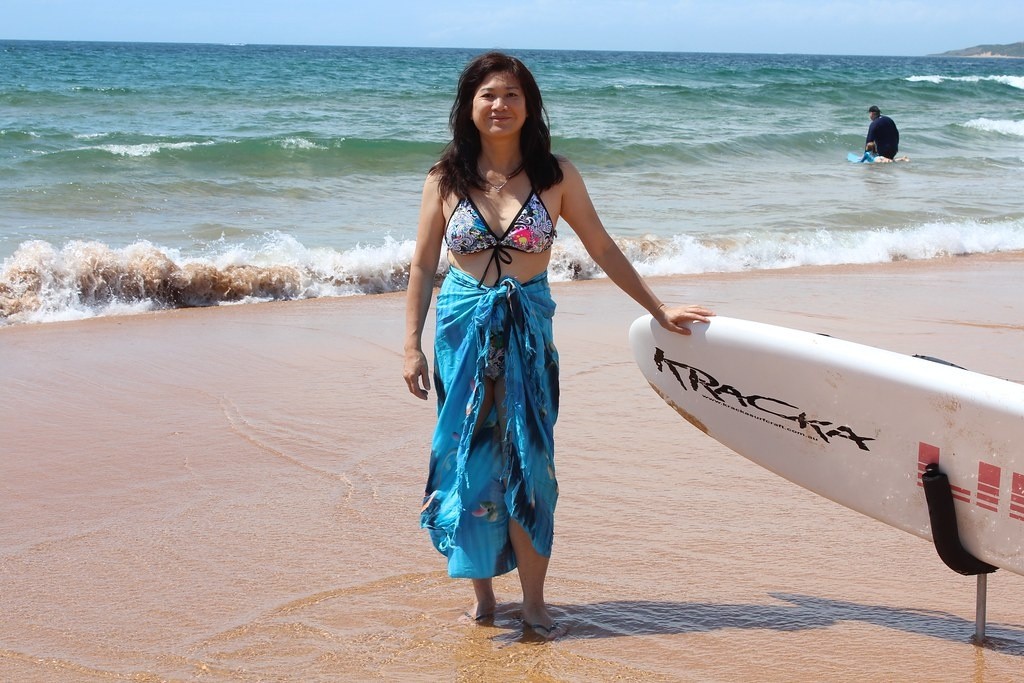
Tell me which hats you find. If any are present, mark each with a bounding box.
[868,105,881,113]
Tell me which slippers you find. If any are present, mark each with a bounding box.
[462,611,494,625]
[522,619,567,641]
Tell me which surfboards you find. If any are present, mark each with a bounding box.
[627,311,1022,575]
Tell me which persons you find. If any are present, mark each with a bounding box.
[861,142,894,162]
[865,106,899,159]
[403,53,714,637]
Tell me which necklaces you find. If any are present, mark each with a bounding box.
[478,159,520,192]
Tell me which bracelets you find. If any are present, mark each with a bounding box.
[652,303,663,316]
